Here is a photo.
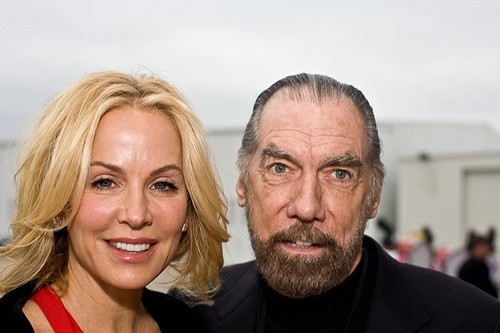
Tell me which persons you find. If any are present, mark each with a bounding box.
[168,72,499,333]
[0,71,230,333]
